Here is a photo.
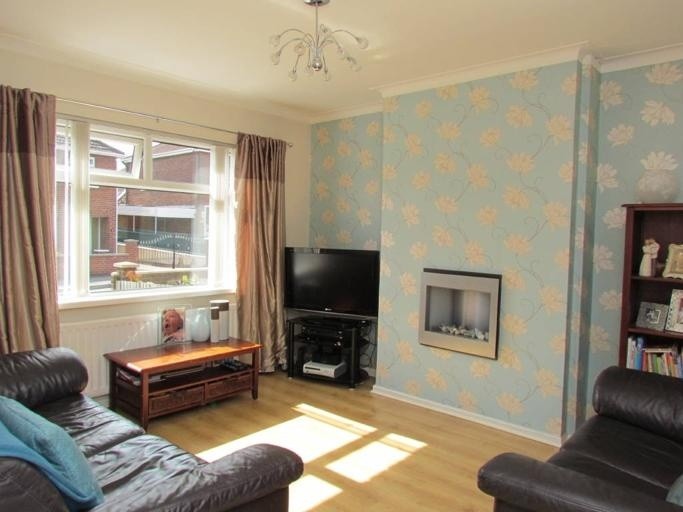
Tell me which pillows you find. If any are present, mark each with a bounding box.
[0,395,106,507]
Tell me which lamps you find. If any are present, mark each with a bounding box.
[267,1,372,84]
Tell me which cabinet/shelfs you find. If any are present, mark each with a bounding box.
[619,202,682,380]
[285,315,370,388]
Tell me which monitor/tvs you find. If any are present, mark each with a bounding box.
[283,246,380,321]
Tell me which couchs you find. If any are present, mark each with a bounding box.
[0,344,305,512]
[476,364,682,510]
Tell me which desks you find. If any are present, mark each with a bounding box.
[103,336,262,434]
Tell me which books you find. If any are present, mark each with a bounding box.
[623,335,682,382]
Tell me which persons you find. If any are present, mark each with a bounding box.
[638,237,654,277]
[646,237,660,277]
[159,309,185,343]
